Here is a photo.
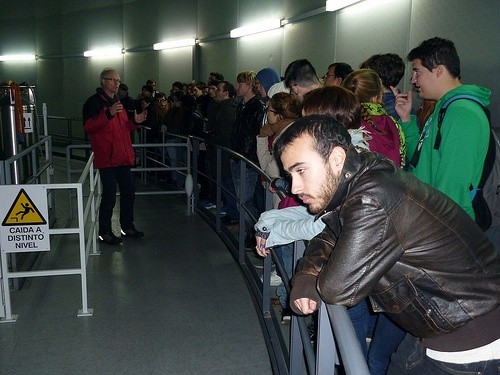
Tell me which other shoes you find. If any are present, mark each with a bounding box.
[244,227,256,251]
[271,298,279,305]
[307,324,317,343]
[202,202,217,208]
[221,214,240,225]
[281,308,292,324]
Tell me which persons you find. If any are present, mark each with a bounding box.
[119,52,406,374]
[81,69,147,243]
[390,38,491,224]
[272,113,500,375]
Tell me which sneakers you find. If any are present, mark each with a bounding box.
[121,228,145,237]
[99,229,122,243]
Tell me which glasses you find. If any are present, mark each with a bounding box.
[103,78,121,83]
[325,74,335,78]
[266,109,283,120]
[290,83,298,97]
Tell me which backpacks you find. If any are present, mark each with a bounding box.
[433,93,500,247]
